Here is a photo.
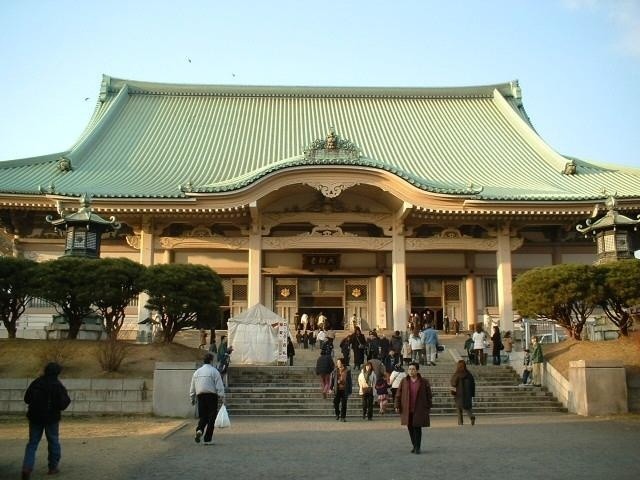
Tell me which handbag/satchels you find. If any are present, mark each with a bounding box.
[363,387,373,394]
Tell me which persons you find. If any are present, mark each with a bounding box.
[284,307,544,453]
[208,339,217,353]
[199,327,207,350]
[190,354,227,445]
[215,335,233,378]
[22,362,72,480]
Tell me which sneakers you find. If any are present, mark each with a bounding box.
[195,430,202,443]
[203,440,216,445]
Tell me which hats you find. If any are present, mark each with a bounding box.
[45,363,63,370]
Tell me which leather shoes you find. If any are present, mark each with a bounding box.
[411,446,420,454]
